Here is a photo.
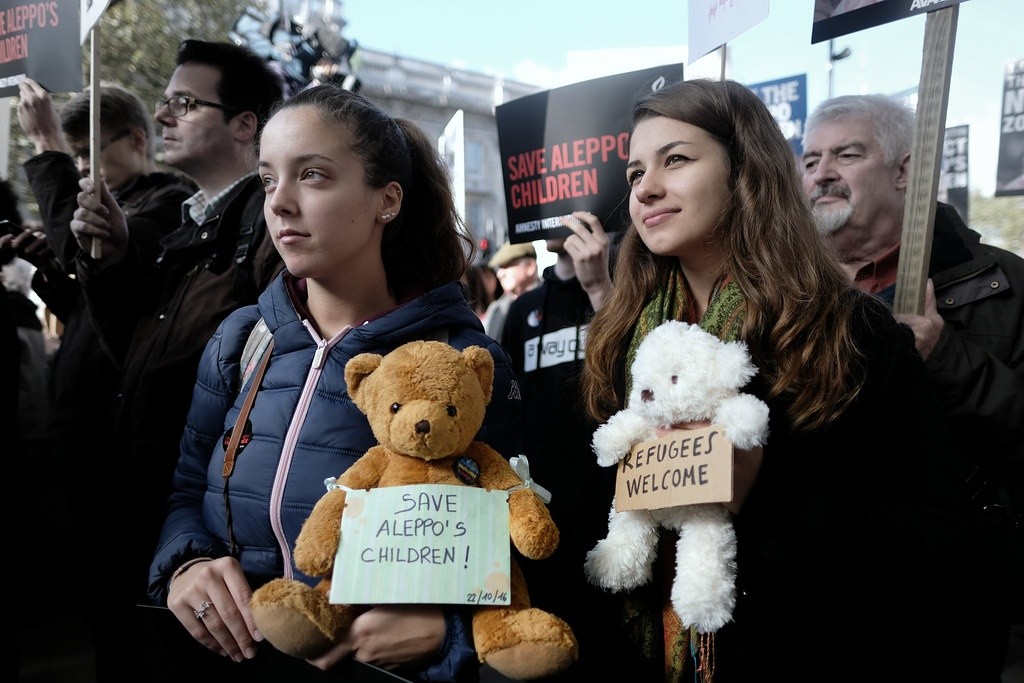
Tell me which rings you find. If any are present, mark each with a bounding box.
[194,601,213,619]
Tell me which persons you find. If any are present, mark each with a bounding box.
[0,38,1024,683]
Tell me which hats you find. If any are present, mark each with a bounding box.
[488,240,536,267]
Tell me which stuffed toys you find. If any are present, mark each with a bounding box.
[584,320,769,634]
[249,339,577,682]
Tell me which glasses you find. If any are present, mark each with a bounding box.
[72,130,131,167]
[155,95,245,117]
